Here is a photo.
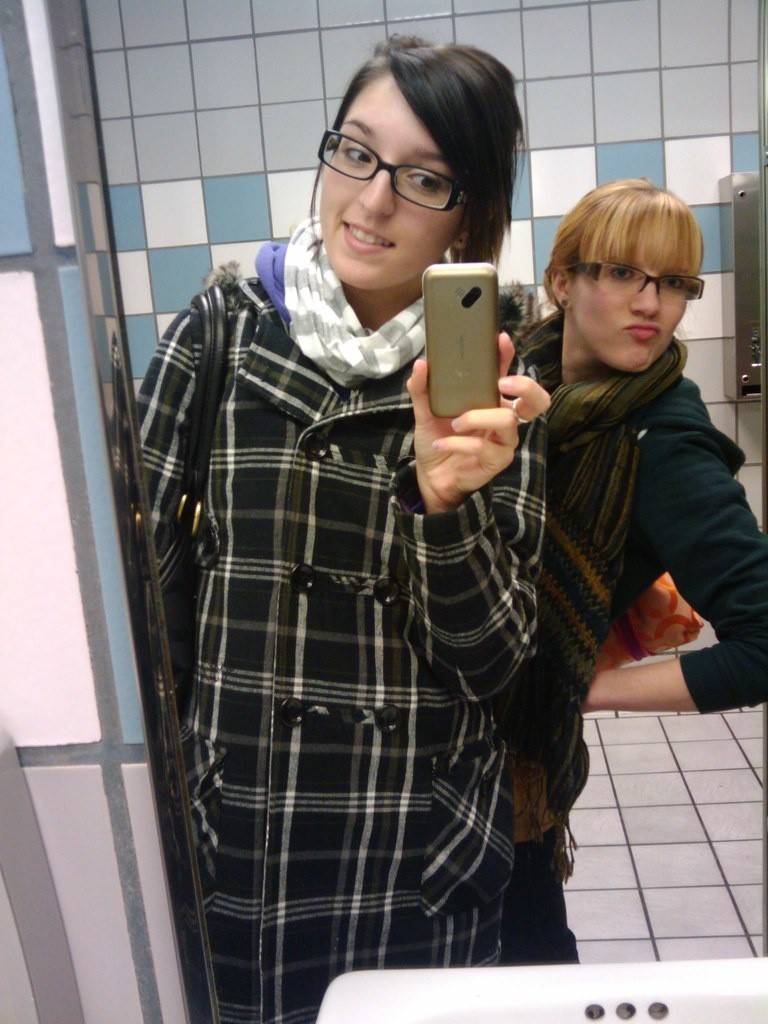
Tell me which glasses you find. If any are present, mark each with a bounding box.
[316,129,464,211]
[571,261,704,300]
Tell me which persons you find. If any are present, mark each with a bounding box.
[500,175,768,967]
[132,33,552,1024]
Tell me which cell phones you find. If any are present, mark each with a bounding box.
[423,263,499,418]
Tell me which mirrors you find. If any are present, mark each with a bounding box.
[47,0,766,1022]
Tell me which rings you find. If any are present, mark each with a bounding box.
[512,397,531,423]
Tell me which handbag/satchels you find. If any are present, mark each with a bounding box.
[595,572,702,676]
[152,287,230,722]
